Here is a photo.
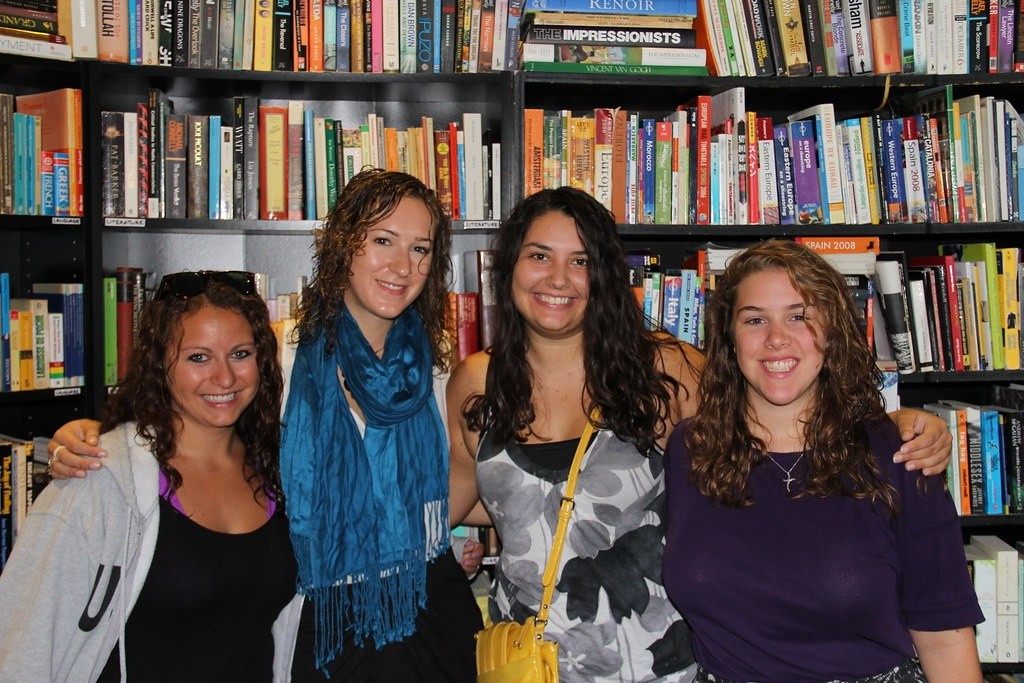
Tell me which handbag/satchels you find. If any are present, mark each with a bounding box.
[477,620,558,683]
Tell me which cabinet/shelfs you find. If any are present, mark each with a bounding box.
[1,1,1024,683]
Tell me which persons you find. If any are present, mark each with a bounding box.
[45,160,499,683]
[439,184,954,683]
[2,268,485,683]
[452,237,989,683]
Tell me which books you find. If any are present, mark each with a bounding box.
[967,532,1018,662]
[1014,551,1024,663]
[0,0,1024,555]
[957,543,996,663]
